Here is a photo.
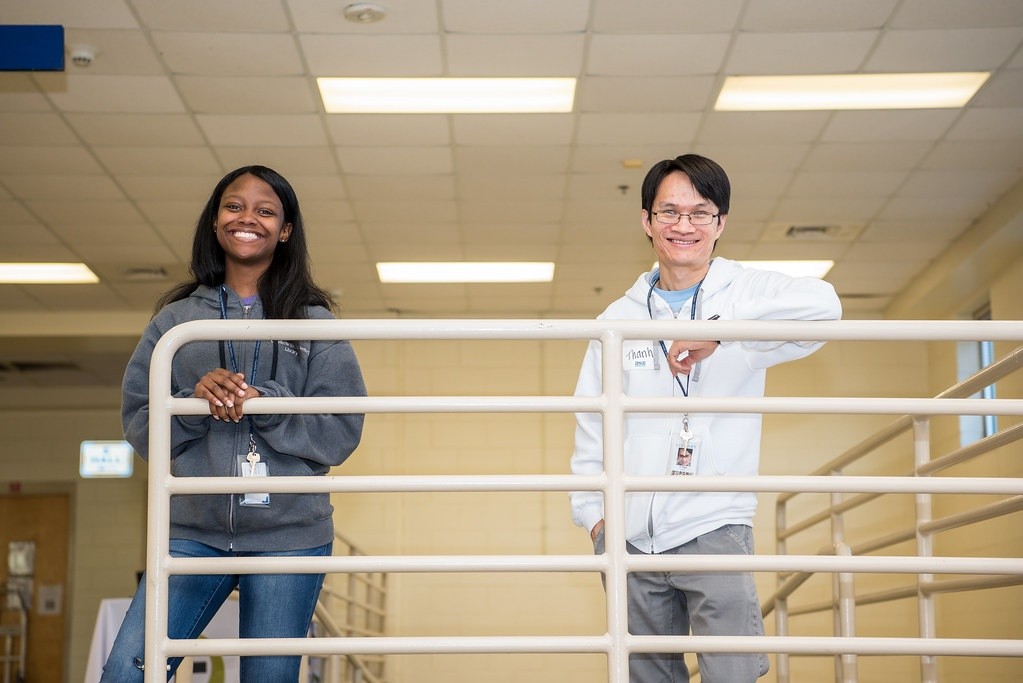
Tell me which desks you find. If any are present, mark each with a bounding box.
[84,596,238,683]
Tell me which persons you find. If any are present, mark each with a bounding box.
[678,448,692,467]
[100,165,368,683]
[568,153,844,683]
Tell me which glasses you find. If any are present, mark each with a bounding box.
[648,209,722,225]
